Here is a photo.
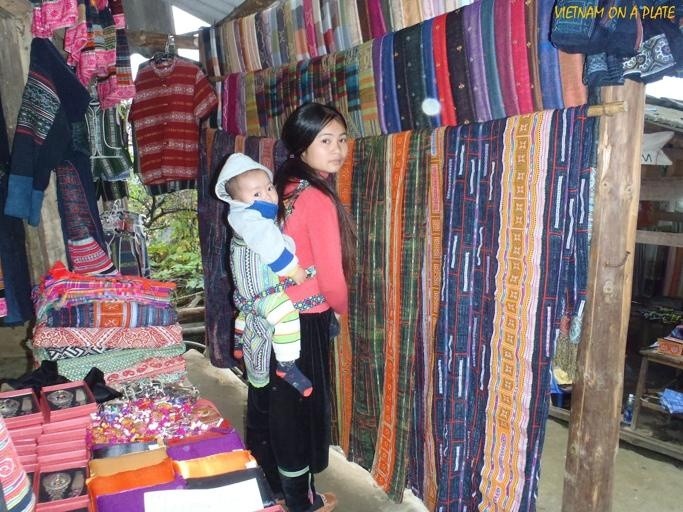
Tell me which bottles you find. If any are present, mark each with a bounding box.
[622,394,634,425]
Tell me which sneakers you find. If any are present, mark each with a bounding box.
[315,492,336,512]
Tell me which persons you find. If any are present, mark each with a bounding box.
[242,99,356,512]
[213,149,316,400]
